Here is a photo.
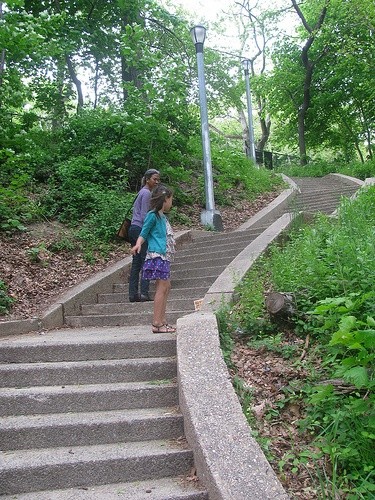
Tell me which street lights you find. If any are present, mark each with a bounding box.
[189,21,224,233]
[240,57,257,167]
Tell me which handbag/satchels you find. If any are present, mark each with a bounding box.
[116,218,132,243]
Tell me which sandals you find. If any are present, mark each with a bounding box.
[152,323,175,333]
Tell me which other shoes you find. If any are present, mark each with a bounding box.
[140,294,154,302]
[130,298,140,302]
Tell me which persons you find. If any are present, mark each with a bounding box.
[129,184,177,334]
[126,168,160,303]
